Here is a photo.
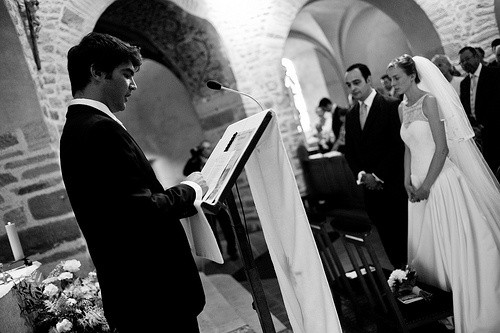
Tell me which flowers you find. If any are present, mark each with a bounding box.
[387,267,434,301]
[0,259,109,333]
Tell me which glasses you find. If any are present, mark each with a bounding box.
[459,54,473,64]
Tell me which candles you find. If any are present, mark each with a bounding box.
[4,221,25,262]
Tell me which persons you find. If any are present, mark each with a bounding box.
[313,38,500,333]
[60,33,210,333]
[183,140,239,260]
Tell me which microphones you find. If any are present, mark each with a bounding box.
[206,80,264,111]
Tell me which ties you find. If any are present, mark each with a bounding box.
[360,102,368,129]
[470,74,477,120]
